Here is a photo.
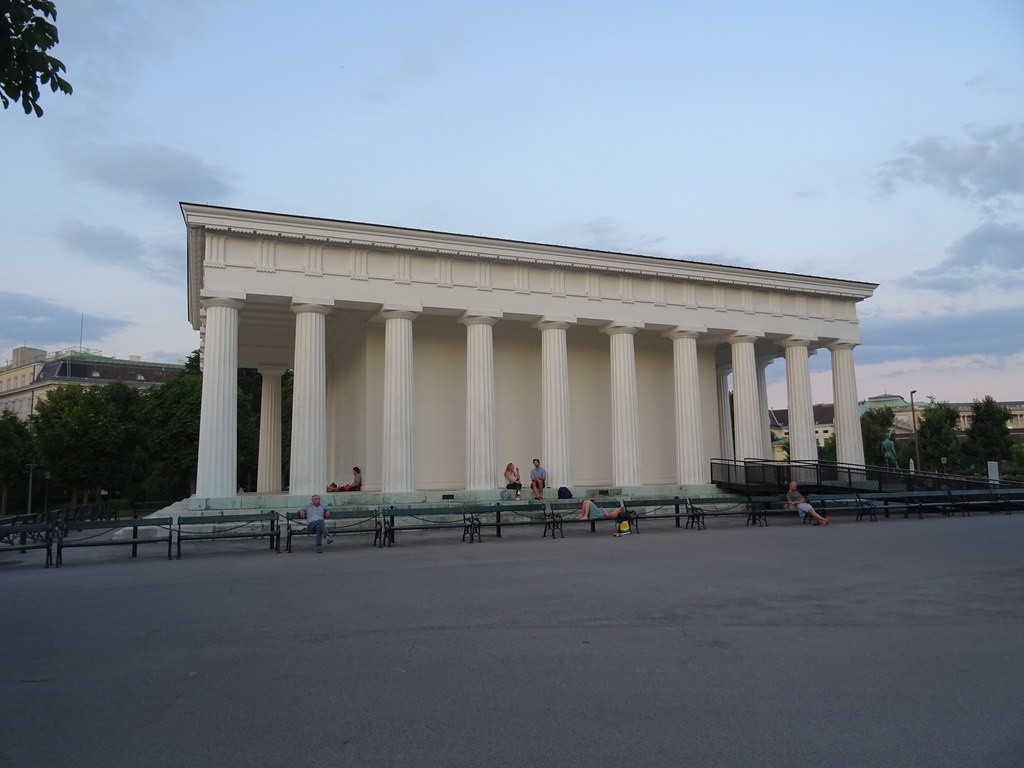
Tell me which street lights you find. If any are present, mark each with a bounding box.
[910,390,922,472]
[44,471,51,515]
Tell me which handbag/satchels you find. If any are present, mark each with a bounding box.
[500,487,512,501]
[558,487,572,499]
[619,518,631,532]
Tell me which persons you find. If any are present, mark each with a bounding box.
[327,466,362,492]
[504,463,522,501]
[530,459,546,501]
[575,499,626,520]
[881,433,899,472]
[299,496,333,553]
[787,482,830,526]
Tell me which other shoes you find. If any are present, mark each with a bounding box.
[515,497,520,500]
[326,538,334,544]
[517,490,520,494]
[315,546,323,553]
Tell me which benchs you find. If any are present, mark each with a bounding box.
[381,501,478,547]
[55,516,173,569]
[688,496,763,530]
[622,497,701,534]
[177,510,281,559]
[468,501,560,542]
[550,499,633,538]
[0,501,118,569]
[753,495,821,527]
[808,487,1024,523]
[285,505,384,554]
